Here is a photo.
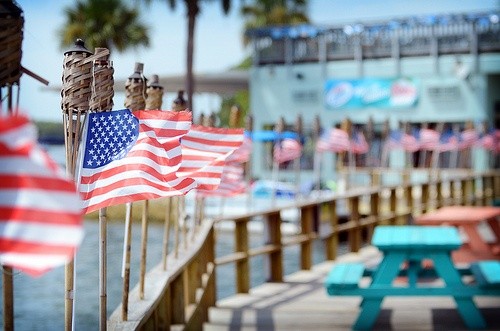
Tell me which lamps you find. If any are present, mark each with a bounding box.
[60,40,93,142]
[91,46,115,115]
[147,74,164,111]
[124,63,148,111]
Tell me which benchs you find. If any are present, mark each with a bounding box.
[326,262,366,289]
[471,260,500,287]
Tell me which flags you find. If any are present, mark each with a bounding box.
[0,114,86,277]
[175,121,500,202]
[73,109,200,214]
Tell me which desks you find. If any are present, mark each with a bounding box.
[352,226,488,331]
[403,206,500,269]
